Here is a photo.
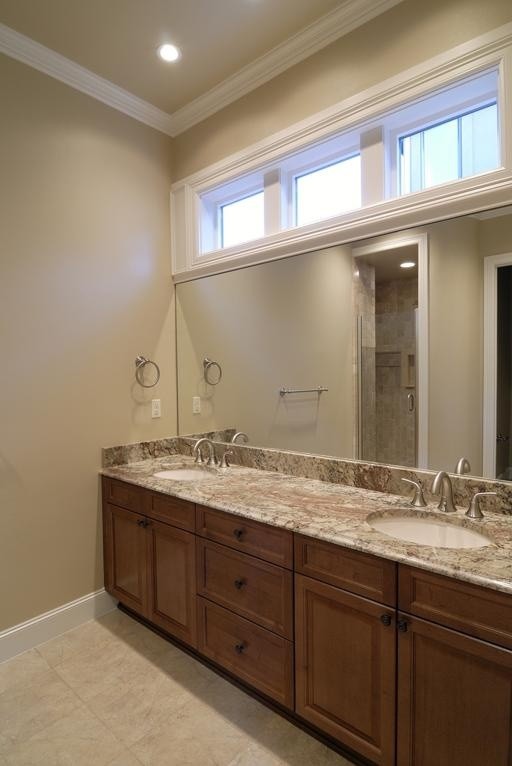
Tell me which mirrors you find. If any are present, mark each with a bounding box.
[174,205,512,484]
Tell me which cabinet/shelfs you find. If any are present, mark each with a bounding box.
[196,504,295,726]
[101,475,196,659]
[294,532,512,766]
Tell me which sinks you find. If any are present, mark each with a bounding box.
[367,508,495,548]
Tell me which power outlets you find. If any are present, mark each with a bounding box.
[152,399,161,419]
[192,397,201,414]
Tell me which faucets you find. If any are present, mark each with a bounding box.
[430,471,457,512]
[230,432,248,443]
[151,462,219,480]
[454,457,471,475]
[193,438,218,465]
